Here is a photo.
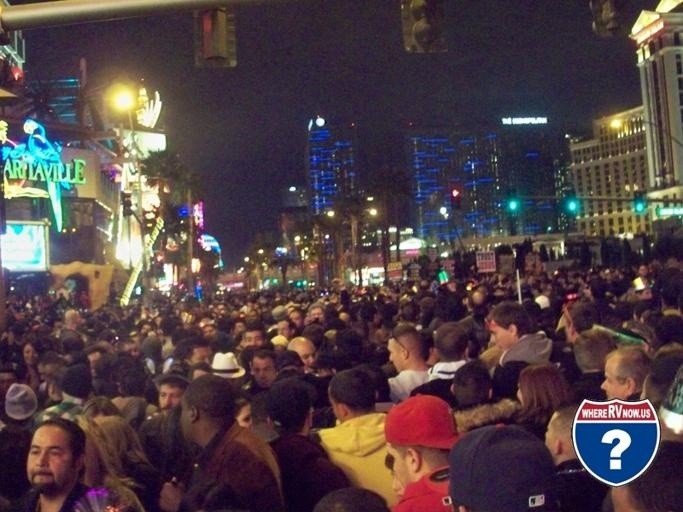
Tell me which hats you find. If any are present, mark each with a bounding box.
[154,367,191,390]
[59,364,93,398]
[272,304,290,321]
[633,276,652,293]
[267,377,317,427]
[448,424,570,511]
[306,350,354,369]
[385,394,465,448]
[5,383,38,420]
[210,351,246,379]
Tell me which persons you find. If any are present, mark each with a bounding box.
[0,258,683,512]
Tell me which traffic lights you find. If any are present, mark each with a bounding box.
[119,189,131,217]
[448,182,461,208]
[141,209,156,234]
[633,188,647,213]
[507,199,520,213]
[562,190,579,216]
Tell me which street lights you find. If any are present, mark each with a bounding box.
[256,248,265,288]
[368,206,388,279]
[293,235,304,279]
[610,118,681,147]
[106,80,152,312]
[242,256,250,291]
[328,208,340,277]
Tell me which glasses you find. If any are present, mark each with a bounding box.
[562,303,573,324]
[483,319,503,330]
[387,328,411,351]
